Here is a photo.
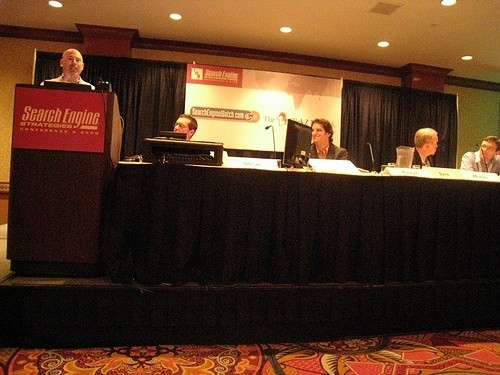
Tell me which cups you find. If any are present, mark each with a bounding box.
[396,146,415,168]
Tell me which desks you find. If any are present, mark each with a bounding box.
[111,162,500,286]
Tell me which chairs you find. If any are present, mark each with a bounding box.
[366,142,378,171]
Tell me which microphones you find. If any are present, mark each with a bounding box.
[476,143,488,172]
[265,125,276,159]
[366,142,374,171]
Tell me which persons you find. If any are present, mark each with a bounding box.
[308,119,348,160]
[461,135,500,175]
[173,114,198,141]
[412,127,439,168]
[41,48,98,90]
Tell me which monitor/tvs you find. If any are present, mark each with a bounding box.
[283,119,312,167]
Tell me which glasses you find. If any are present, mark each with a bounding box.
[173,122,189,129]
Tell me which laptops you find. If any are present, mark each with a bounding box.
[44,80,91,91]
